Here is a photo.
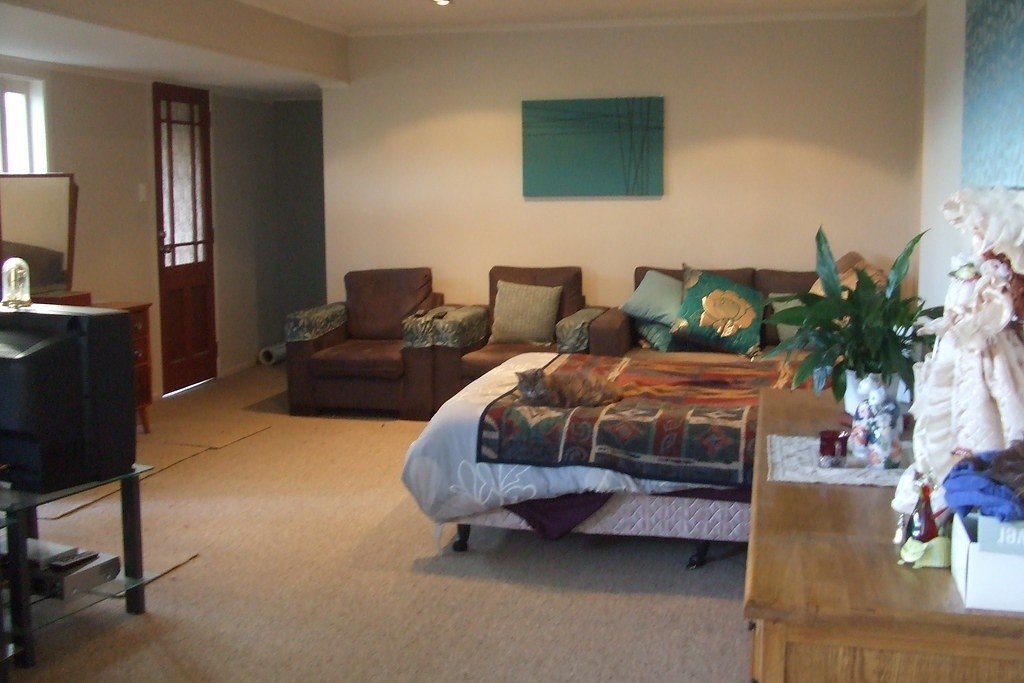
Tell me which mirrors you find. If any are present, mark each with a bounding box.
[0,173,78,303]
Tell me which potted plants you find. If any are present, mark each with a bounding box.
[759,226,945,417]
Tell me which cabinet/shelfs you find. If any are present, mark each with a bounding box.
[33,291,154,434]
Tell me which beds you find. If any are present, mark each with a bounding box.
[401,351,845,569]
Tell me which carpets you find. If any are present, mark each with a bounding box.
[36,417,273,519]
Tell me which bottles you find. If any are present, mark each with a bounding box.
[906,486,938,544]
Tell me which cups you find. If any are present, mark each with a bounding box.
[818,430,851,467]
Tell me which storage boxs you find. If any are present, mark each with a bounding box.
[950,513,1024,612]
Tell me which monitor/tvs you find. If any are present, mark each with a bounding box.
[0,301,137,495]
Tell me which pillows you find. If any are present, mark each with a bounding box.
[808,251,887,329]
[486,280,563,347]
[768,292,820,342]
[632,318,682,353]
[619,269,683,328]
[668,263,766,358]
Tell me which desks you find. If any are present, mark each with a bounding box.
[743,386,1024,683]
[0,464,158,683]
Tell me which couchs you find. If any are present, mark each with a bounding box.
[433,266,610,415]
[588,265,883,359]
[284,268,464,422]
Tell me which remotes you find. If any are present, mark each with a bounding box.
[51,549,99,571]
